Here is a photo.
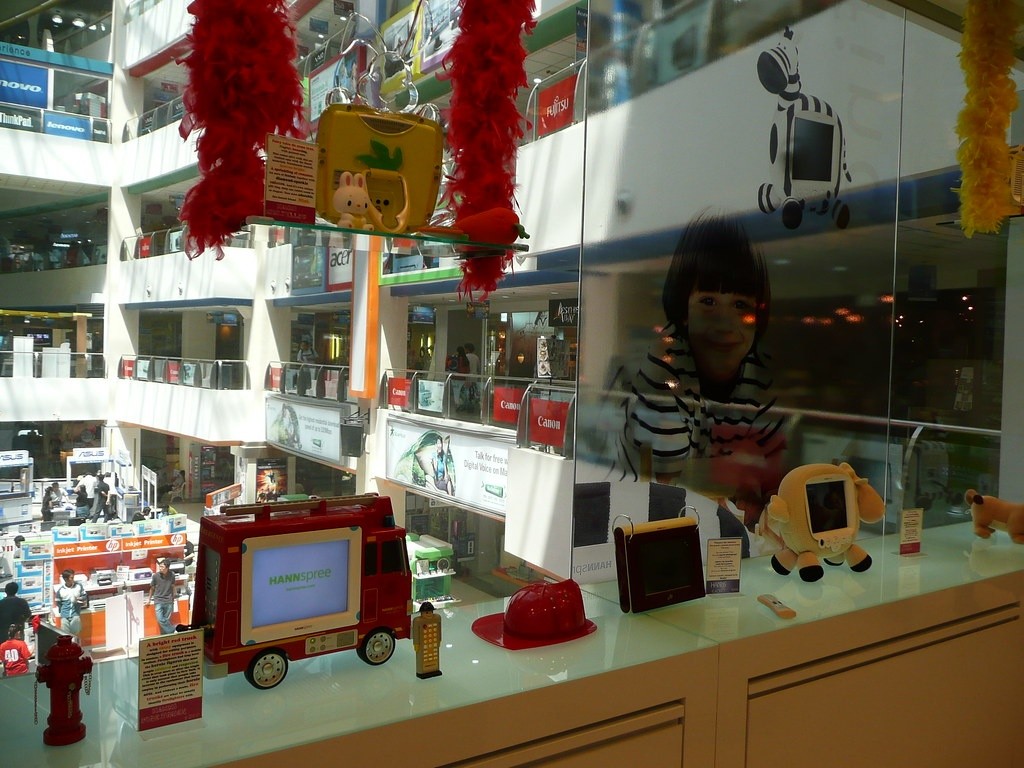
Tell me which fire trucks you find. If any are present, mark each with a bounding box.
[174,489,413,690]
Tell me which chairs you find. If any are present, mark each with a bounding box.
[168,482,186,503]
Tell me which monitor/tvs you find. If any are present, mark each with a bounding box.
[411,304,434,325]
[220,313,238,326]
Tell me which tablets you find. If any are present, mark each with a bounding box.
[239,526,362,647]
[625,525,707,614]
[803,474,856,542]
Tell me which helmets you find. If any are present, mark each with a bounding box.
[471,578,597,650]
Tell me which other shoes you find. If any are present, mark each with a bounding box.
[456,405,460,412]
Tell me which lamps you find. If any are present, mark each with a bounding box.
[73,18,86,27]
[51,14,63,23]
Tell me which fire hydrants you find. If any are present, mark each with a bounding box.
[34,637,94,746]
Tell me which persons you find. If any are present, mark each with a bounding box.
[448,343,480,414]
[0,583,37,676]
[184,539,196,583]
[40,469,119,527]
[143,558,179,635]
[47,431,65,477]
[161,504,179,518]
[154,469,186,503]
[130,507,151,524]
[296,339,319,369]
[55,568,86,643]
